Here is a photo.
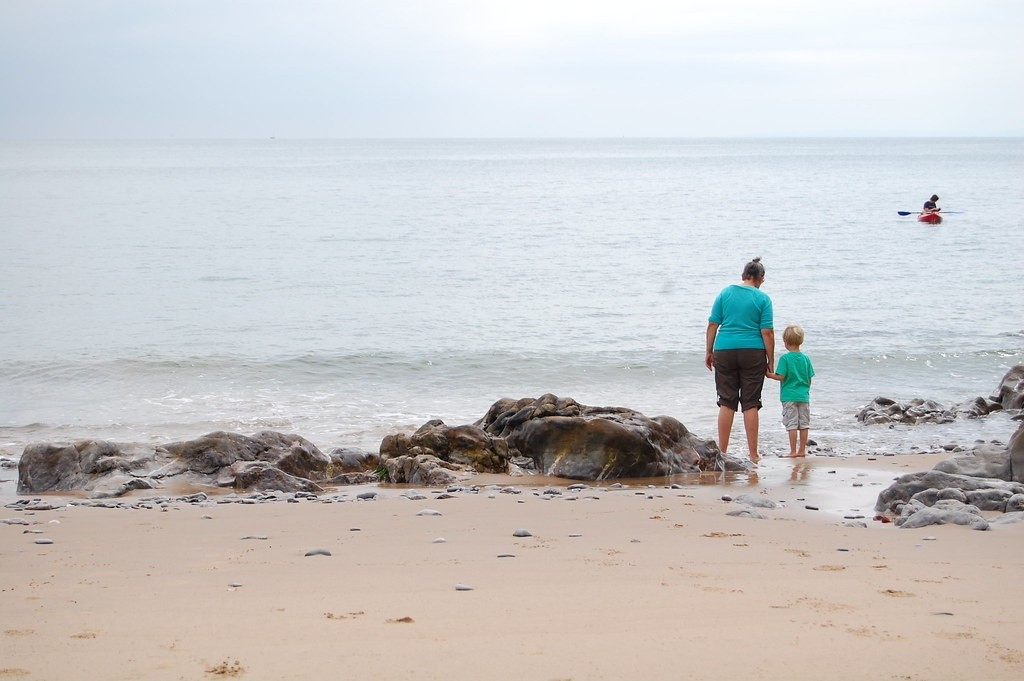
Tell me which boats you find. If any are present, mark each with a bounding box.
[917,213,943,224]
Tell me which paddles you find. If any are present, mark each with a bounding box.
[898,211,962,216]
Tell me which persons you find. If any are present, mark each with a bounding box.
[921,195,941,215]
[765,325,815,458]
[705,256,775,461]
